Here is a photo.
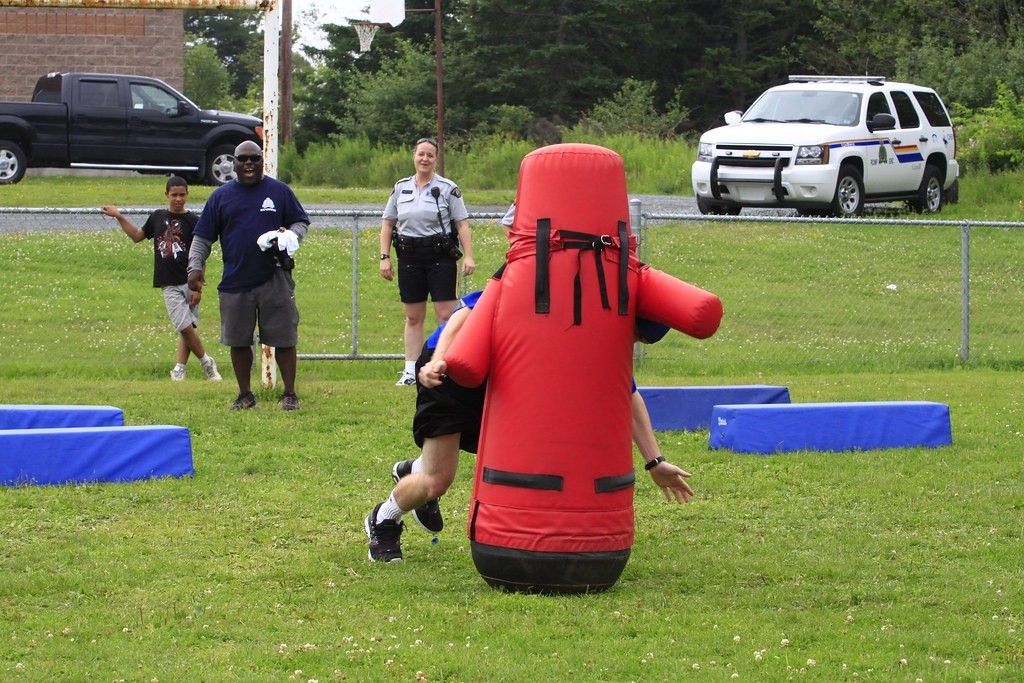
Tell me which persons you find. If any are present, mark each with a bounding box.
[502,199,517,236]
[365,291,695,565]
[186,140,311,410]
[99,176,222,381]
[379,138,476,387]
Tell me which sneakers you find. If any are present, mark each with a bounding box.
[230,391,256,410]
[201,356,222,380]
[170,364,187,381]
[364,501,407,564]
[393,460,443,533]
[395,369,418,387]
[280,391,300,410]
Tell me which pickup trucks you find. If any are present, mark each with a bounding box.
[0,68,265,186]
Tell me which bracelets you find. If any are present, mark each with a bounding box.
[645,456,665,471]
[380,254,389,260]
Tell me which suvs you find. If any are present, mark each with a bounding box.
[690,72,960,218]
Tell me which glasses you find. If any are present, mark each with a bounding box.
[234,154,262,162]
[416,138,438,148]
